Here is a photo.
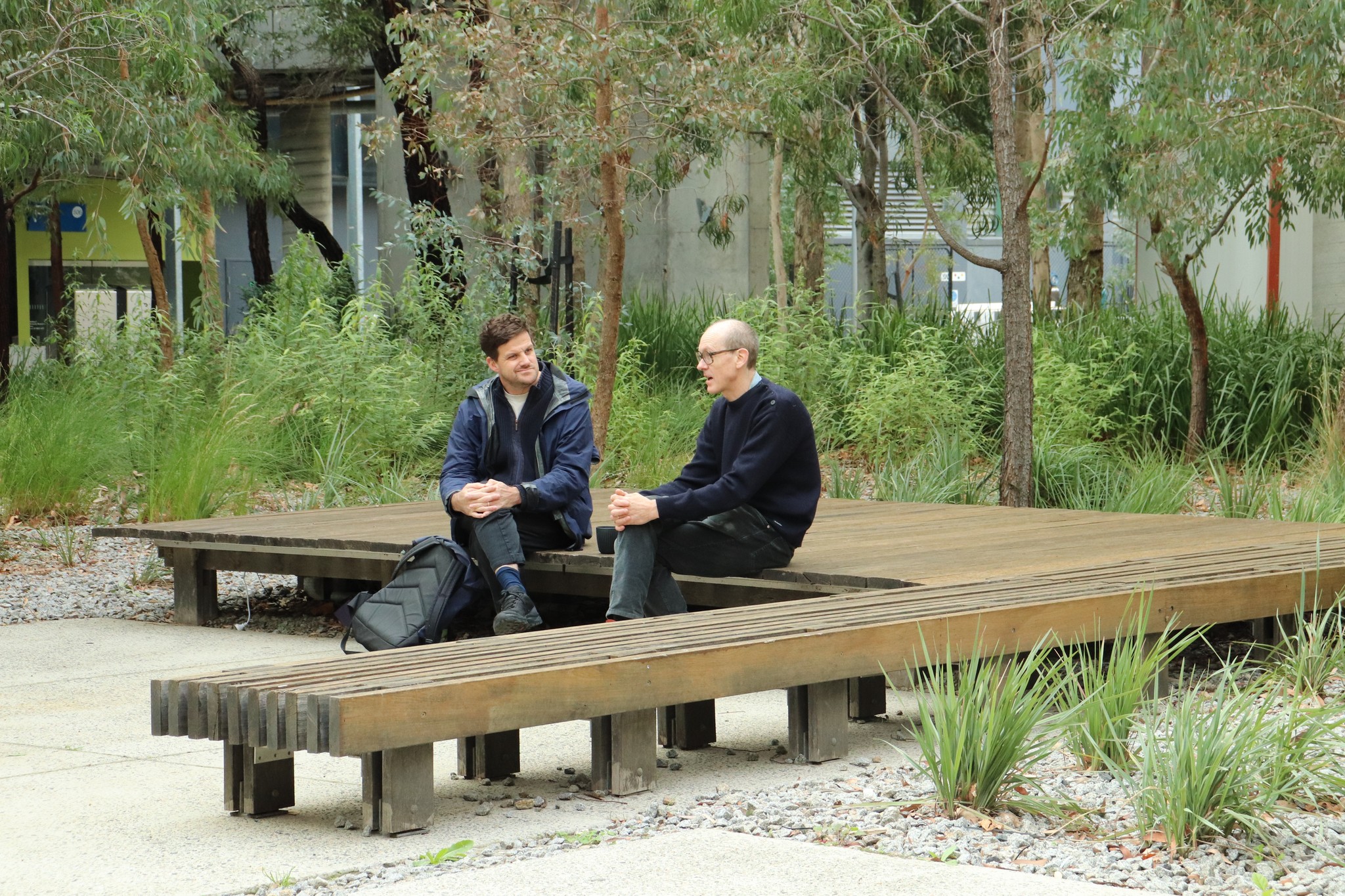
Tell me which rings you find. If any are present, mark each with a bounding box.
[488,502,489,506]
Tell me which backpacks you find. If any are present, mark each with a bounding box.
[350,536,472,652]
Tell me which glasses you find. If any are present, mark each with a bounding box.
[695,349,738,365]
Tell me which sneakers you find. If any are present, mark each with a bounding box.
[493,586,543,635]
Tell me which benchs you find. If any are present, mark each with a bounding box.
[88,486,1345,835]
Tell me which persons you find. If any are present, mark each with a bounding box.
[440,313,600,635]
[606,319,821,622]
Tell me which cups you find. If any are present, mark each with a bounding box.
[596,526,618,554]
